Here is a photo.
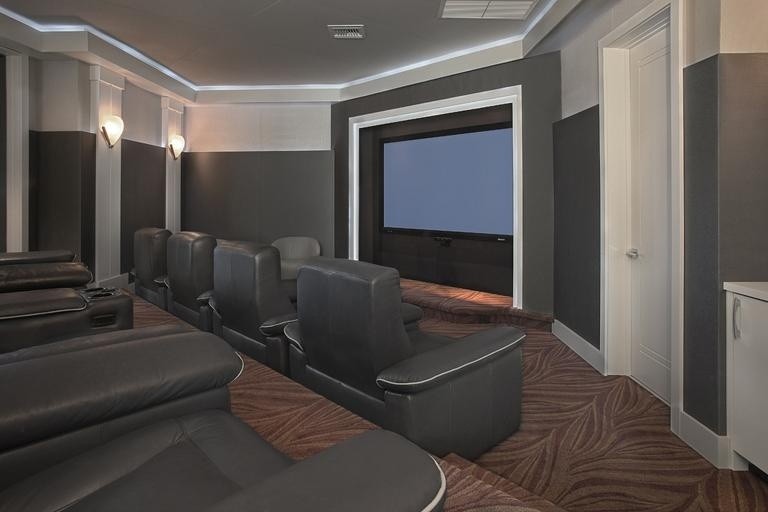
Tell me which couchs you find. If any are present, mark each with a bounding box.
[133,229,526,461]
[1,248,446,512]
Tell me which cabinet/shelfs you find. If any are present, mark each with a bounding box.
[723,282,768,477]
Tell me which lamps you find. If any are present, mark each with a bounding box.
[169,135,185,160]
[102,116,124,148]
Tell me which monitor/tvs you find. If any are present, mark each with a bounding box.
[377,121,511,244]
